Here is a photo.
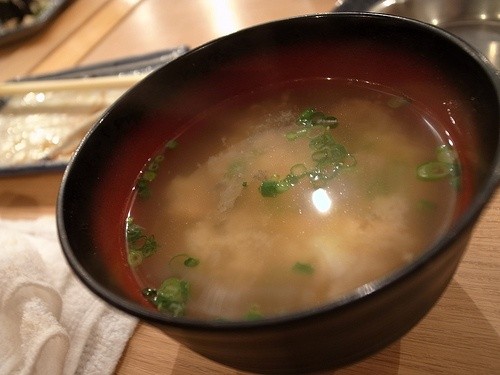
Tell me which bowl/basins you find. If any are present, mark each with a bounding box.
[55,10,500,374]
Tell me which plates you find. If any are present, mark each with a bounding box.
[0,43,192,171]
[322,0,500,74]
[0,1,64,46]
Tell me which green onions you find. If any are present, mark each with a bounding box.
[124,107,463,323]
